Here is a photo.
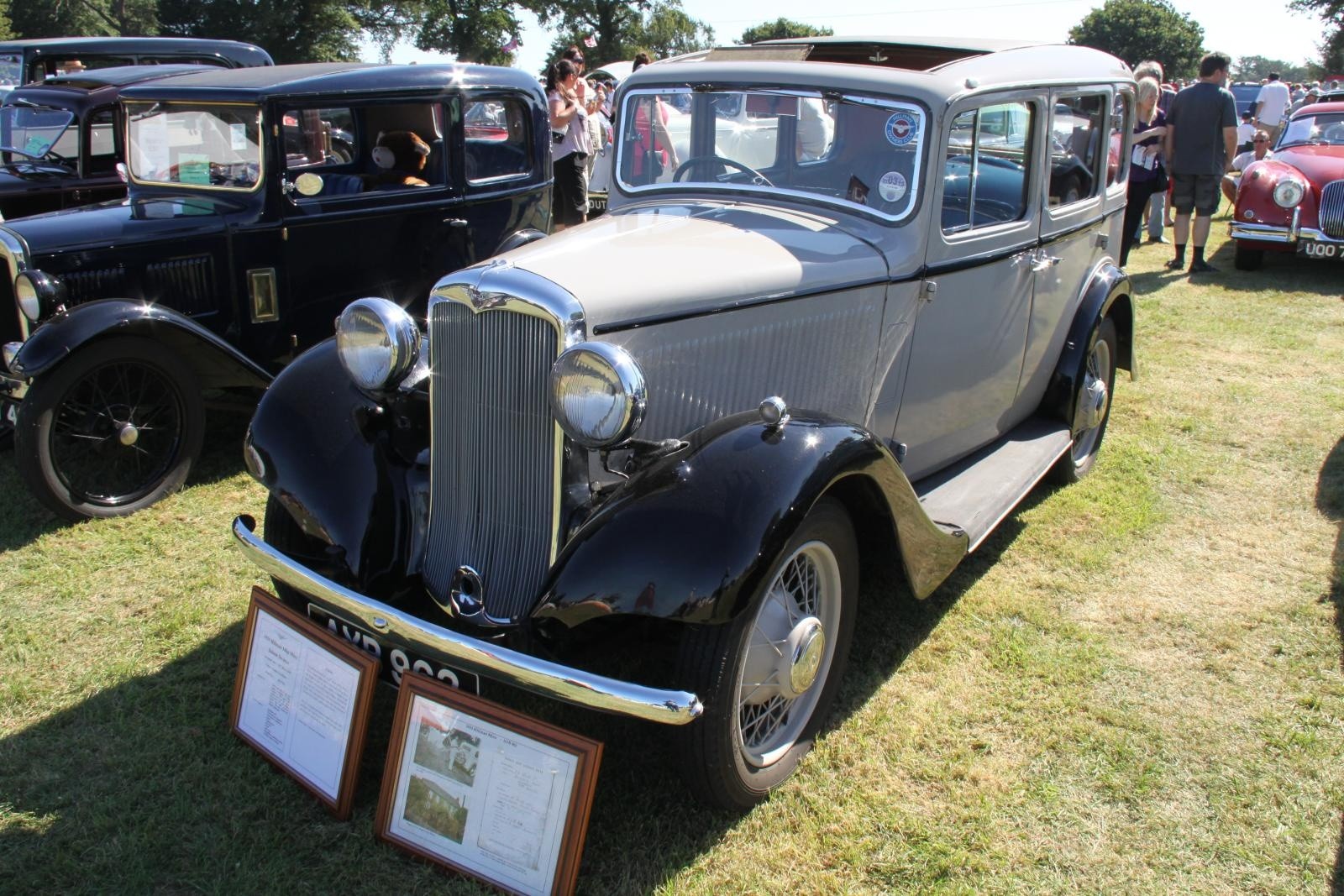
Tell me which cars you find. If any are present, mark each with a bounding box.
[901,103,1123,204]
[231,30,1136,805]
[1229,91,1344,271]
[0,35,553,521]
[569,54,835,220]
[460,91,512,143]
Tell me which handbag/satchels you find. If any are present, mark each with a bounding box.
[1153,161,1170,192]
[642,150,663,176]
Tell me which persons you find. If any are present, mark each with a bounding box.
[1251,71,1292,150]
[62,60,87,74]
[1108,51,1340,274]
[1221,128,1272,205]
[1164,50,1238,274]
[795,97,835,162]
[545,44,679,232]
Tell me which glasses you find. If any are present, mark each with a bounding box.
[1253,140,1265,143]
[570,58,584,64]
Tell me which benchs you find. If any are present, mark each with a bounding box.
[426,137,530,187]
[796,181,998,231]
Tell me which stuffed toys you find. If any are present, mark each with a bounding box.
[372,131,431,187]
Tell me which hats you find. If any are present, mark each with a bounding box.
[1308,89,1321,96]
[1241,111,1251,120]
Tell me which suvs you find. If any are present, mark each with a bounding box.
[1227,81,1263,115]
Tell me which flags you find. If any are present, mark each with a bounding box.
[584,33,598,47]
[499,34,517,54]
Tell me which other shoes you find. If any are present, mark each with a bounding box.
[1148,235,1171,244]
[1188,261,1221,273]
[1166,259,1183,269]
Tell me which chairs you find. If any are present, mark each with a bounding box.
[299,171,366,194]
